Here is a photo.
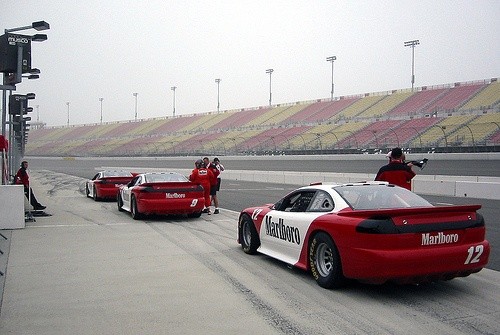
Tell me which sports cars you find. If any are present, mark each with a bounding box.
[117,172,206,219]
[236,180,491,289]
[85,170,134,201]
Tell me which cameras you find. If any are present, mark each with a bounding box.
[214,161,217,163]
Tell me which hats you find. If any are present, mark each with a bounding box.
[385,151,392,158]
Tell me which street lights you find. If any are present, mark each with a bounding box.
[215,79,221,112]
[133,93,138,123]
[65,102,70,126]
[404,40,420,91]
[326,56,336,99]
[99,97,104,123]
[0,20,50,185]
[170,86,177,116]
[266,69,274,105]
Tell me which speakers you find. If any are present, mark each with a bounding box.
[13,116,21,131]
[9,95,28,115]
[0,33,31,73]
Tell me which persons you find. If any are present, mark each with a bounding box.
[210,158,224,206]
[15,161,47,210]
[189,160,204,181]
[191,162,217,215]
[374,147,416,191]
[202,157,219,214]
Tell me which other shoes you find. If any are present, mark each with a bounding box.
[214,210,219,214]
[202,208,209,213]
[208,210,212,215]
[33,203,46,210]
